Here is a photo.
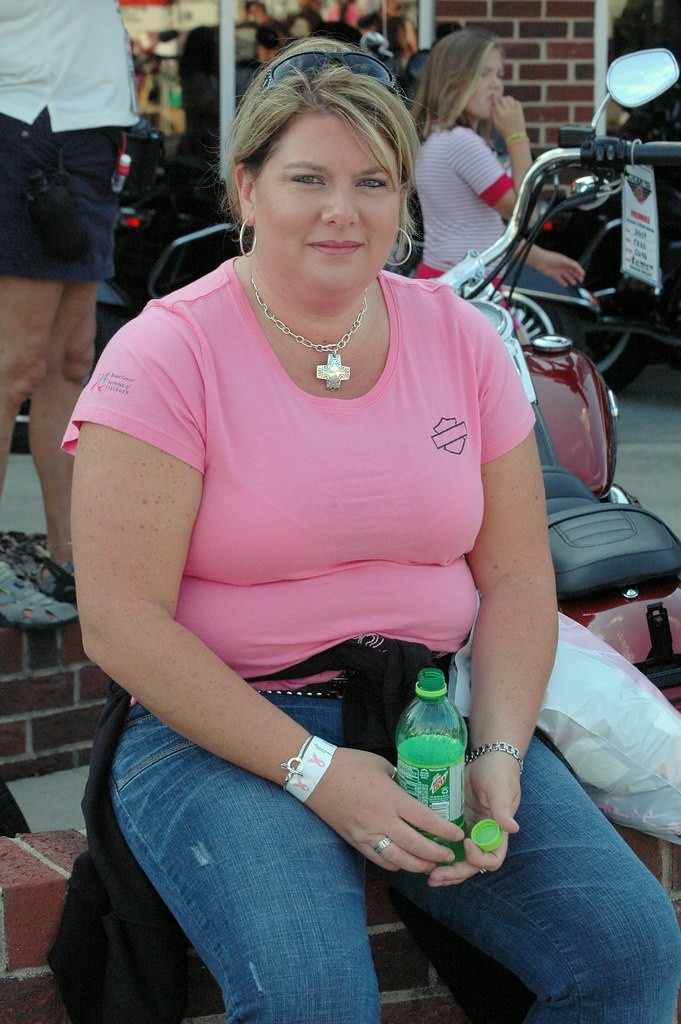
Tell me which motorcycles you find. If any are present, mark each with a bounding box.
[399,47,681,716]
[8,21,681,456]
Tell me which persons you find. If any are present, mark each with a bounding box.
[130,0,584,345]
[61,37,681,1024]
[0,0,140,631]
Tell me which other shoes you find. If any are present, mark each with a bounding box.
[38,563,77,601]
[0,562,77,627]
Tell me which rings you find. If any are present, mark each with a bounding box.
[479,867,486,873]
[374,838,393,853]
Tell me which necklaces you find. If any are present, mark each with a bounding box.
[251,278,368,391]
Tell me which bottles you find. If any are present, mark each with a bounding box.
[397,668,468,874]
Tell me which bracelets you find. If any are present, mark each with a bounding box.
[280,736,337,804]
[505,133,529,154]
[464,743,523,778]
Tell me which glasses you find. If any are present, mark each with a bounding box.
[260,50,400,96]
